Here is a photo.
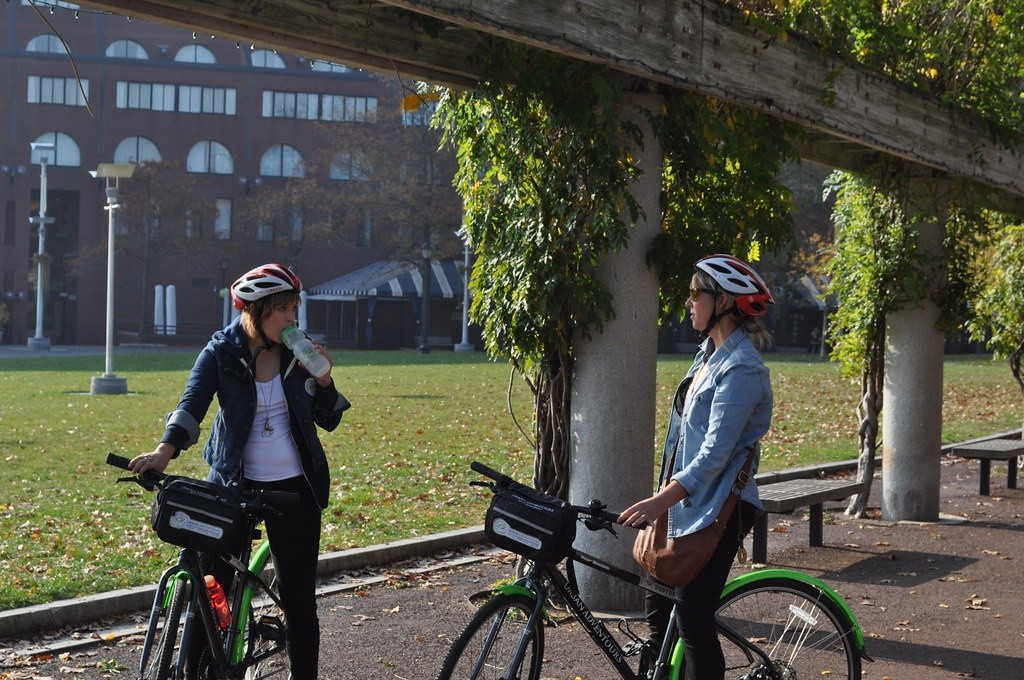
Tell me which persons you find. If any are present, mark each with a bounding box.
[128,263,351,680]
[617,254,773,680]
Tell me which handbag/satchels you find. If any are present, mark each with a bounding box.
[632,478,739,586]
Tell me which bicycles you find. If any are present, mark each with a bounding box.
[104,450,294,680]
[439,460,876,680]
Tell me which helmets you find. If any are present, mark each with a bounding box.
[694,254,776,315]
[230,263,303,310]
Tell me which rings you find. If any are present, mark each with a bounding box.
[145,456,153,462]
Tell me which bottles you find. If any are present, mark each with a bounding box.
[203,575,233,627]
[280,324,331,377]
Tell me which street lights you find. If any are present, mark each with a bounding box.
[90,162,138,394]
[28,142,57,349]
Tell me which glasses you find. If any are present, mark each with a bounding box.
[689,287,725,302]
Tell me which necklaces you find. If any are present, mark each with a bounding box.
[252,342,279,437]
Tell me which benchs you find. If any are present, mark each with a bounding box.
[415,335,455,349]
[953,439,1024,495]
[752,478,864,565]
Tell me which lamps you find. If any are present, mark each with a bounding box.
[6,292,25,303]
[0,164,27,183]
[238,176,262,194]
